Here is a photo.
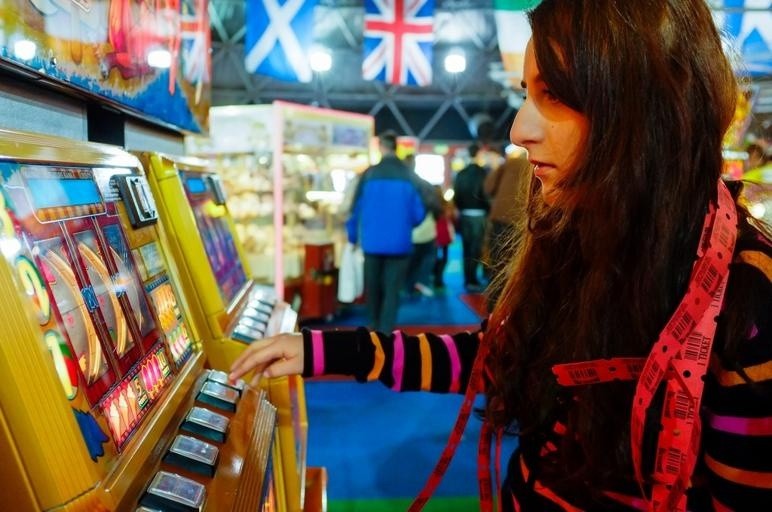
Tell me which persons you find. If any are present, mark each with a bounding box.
[344,129,535,335]
[224,0,772,512]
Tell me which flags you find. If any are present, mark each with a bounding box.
[167,0,216,86]
[241,0,317,84]
[363,0,439,87]
